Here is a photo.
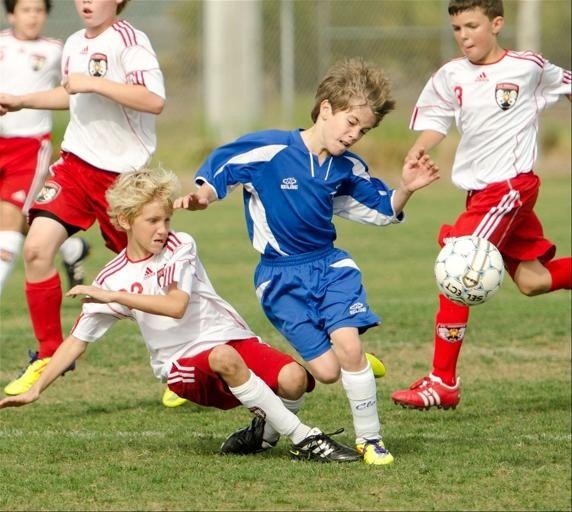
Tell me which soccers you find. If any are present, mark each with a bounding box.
[435,236,505,306]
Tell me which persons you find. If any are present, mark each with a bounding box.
[387,0,571,410]
[172,60,440,467]
[0,164,364,462]
[2,0,187,408]
[1,0,90,298]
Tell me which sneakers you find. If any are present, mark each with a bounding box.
[63,235,90,298]
[365,351,386,378]
[287,427,363,463]
[220,416,279,456]
[354,439,394,466]
[392,373,461,410]
[162,387,187,409]
[3,349,75,398]
